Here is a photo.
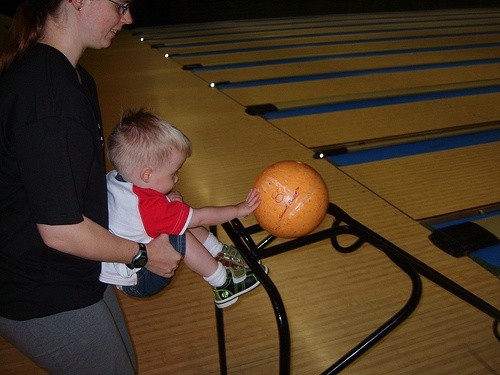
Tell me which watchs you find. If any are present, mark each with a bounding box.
[125,242,148,270]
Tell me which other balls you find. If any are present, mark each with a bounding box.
[250,160,329,240]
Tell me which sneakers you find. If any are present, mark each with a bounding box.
[214,244,261,270]
[211,264,268,308]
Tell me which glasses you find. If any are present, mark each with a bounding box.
[108,0,131,15]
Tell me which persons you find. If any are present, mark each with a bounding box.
[98,108,269,308]
[0,0,133,375]
